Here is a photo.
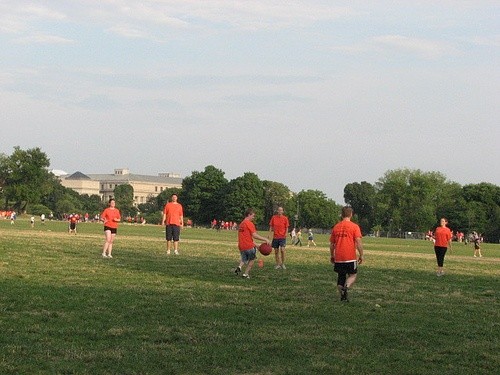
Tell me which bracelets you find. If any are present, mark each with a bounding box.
[359,256,363,259]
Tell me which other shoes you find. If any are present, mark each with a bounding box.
[274,264,286,270]
[101,253,109,258]
[174,250,179,255]
[107,254,114,258]
[167,250,170,255]
[340,288,349,303]
[473,255,477,258]
[235,265,241,275]
[480,254,482,257]
[242,273,250,279]
[436,271,444,276]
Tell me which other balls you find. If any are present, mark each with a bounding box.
[259,243,271,255]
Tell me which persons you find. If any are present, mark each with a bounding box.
[30,214,34,228]
[48,211,105,224]
[432,215,453,278]
[208,217,238,232]
[40,213,45,225]
[295,228,303,248]
[0,207,27,225]
[266,204,290,270]
[234,208,270,279]
[376,229,381,240]
[329,206,364,302]
[306,228,317,248]
[424,229,483,244]
[290,227,297,246]
[68,212,78,235]
[122,209,146,225]
[99,198,121,259]
[186,218,193,229]
[162,194,184,255]
[473,238,483,258]
